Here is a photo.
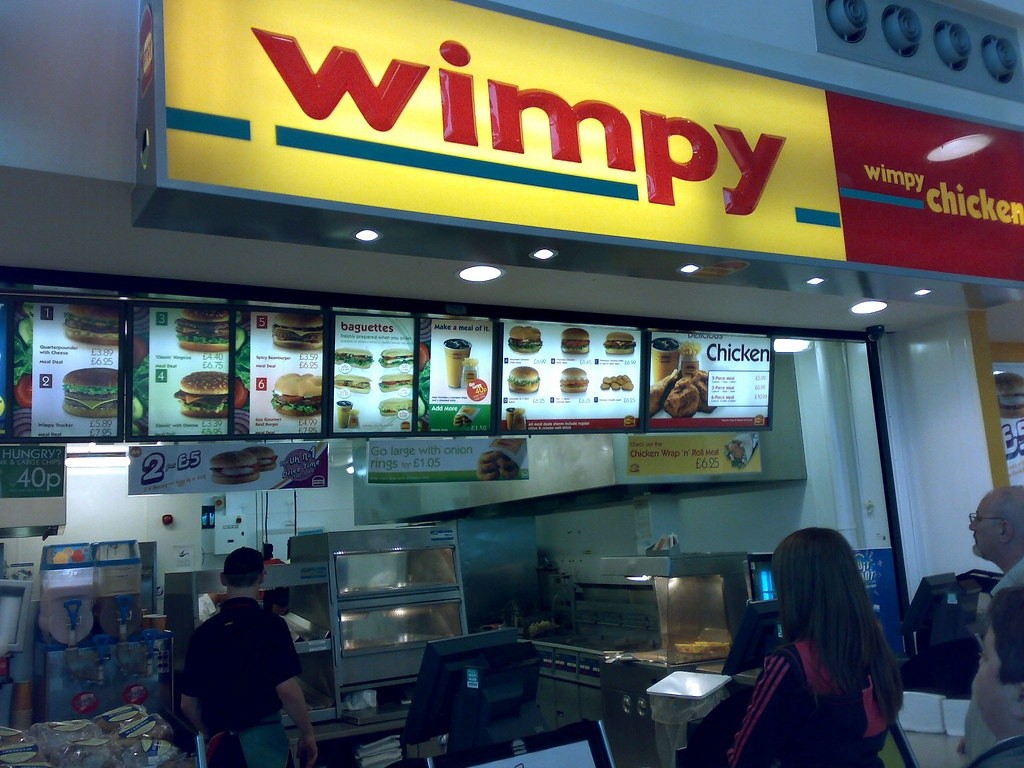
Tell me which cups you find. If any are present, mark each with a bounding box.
[506,408,515,430]
[443,338,472,388]
[651,337,681,382]
[335,400,352,428]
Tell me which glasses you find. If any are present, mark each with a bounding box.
[969,512,1004,527]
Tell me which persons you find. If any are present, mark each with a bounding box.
[955,487,1024,768]
[726,528,903,768]
[180,542,318,768]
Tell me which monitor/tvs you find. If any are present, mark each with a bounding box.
[399,628,521,750]
[428,720,616,768]
[721,598,782,676]
[901,573,957,636]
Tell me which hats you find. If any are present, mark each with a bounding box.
[223,547,266,577]
